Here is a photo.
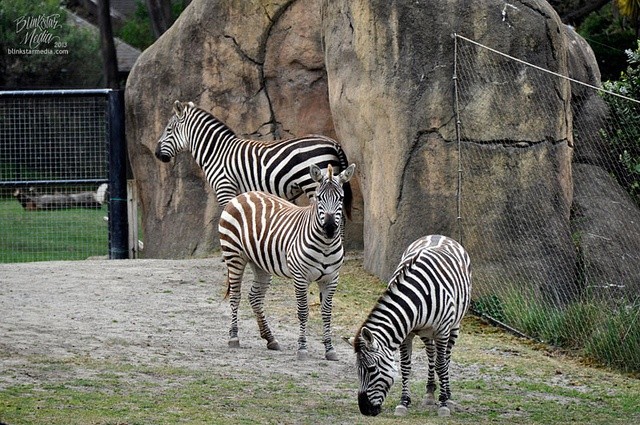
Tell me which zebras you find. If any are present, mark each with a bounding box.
[216,162,357,362]
[339,232,475,418]
[153,98,355,265]
